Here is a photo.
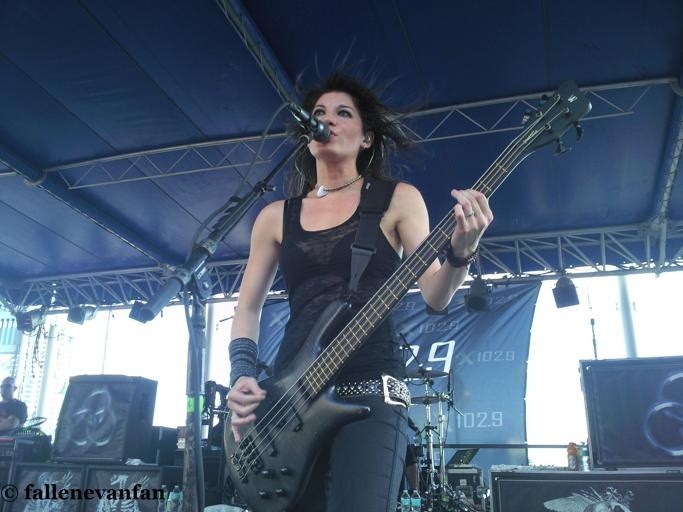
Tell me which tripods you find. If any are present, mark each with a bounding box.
[396,381,479,512]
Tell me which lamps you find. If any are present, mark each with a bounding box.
[462,274,492,315]
[66,304,100,325]
[128,300,149,326]
[552,273,580,309]
[16,308,43,333]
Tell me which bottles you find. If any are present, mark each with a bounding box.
[400,489,422,512]
[567,441,591,472]
[157,485,184,512]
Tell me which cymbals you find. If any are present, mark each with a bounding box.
[404,371,448,379]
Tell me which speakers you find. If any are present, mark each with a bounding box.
[79,465,163,512]
[50,375,158,465]
[580,356,683,469]
[491,471,683,512]
[5,463,85,512]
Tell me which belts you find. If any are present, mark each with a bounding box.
[335,371,411,409]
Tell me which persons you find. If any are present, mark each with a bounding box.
[0,376,28,437]
[223,66,497,510]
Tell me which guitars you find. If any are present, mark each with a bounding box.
[224,78,590,509]
[411,397,445,403]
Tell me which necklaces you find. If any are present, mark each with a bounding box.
[314,174,363,198]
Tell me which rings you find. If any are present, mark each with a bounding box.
[465,212,475,218]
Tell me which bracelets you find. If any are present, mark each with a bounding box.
[445,245,480,271]
[228,337,259,389]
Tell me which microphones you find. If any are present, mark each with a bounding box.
[448,372,450,392]
[288,102,330,143]
